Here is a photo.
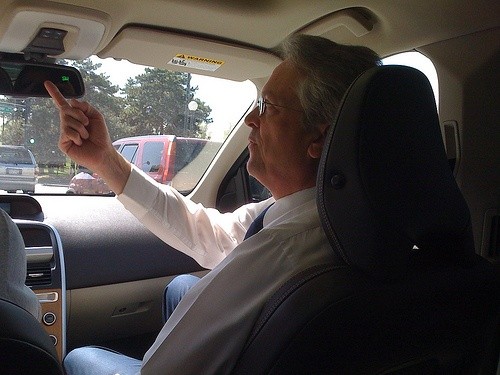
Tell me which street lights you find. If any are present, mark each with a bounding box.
[187,101,198,138]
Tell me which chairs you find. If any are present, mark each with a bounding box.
[230,63,500,375]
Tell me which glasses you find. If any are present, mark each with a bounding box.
[257,96,311,117]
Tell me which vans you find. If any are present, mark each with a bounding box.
[64,134,223,207]
[0,143,40,195]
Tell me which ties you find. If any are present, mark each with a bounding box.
[240,203,278,241]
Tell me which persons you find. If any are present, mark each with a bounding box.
[43,35,384,375]
